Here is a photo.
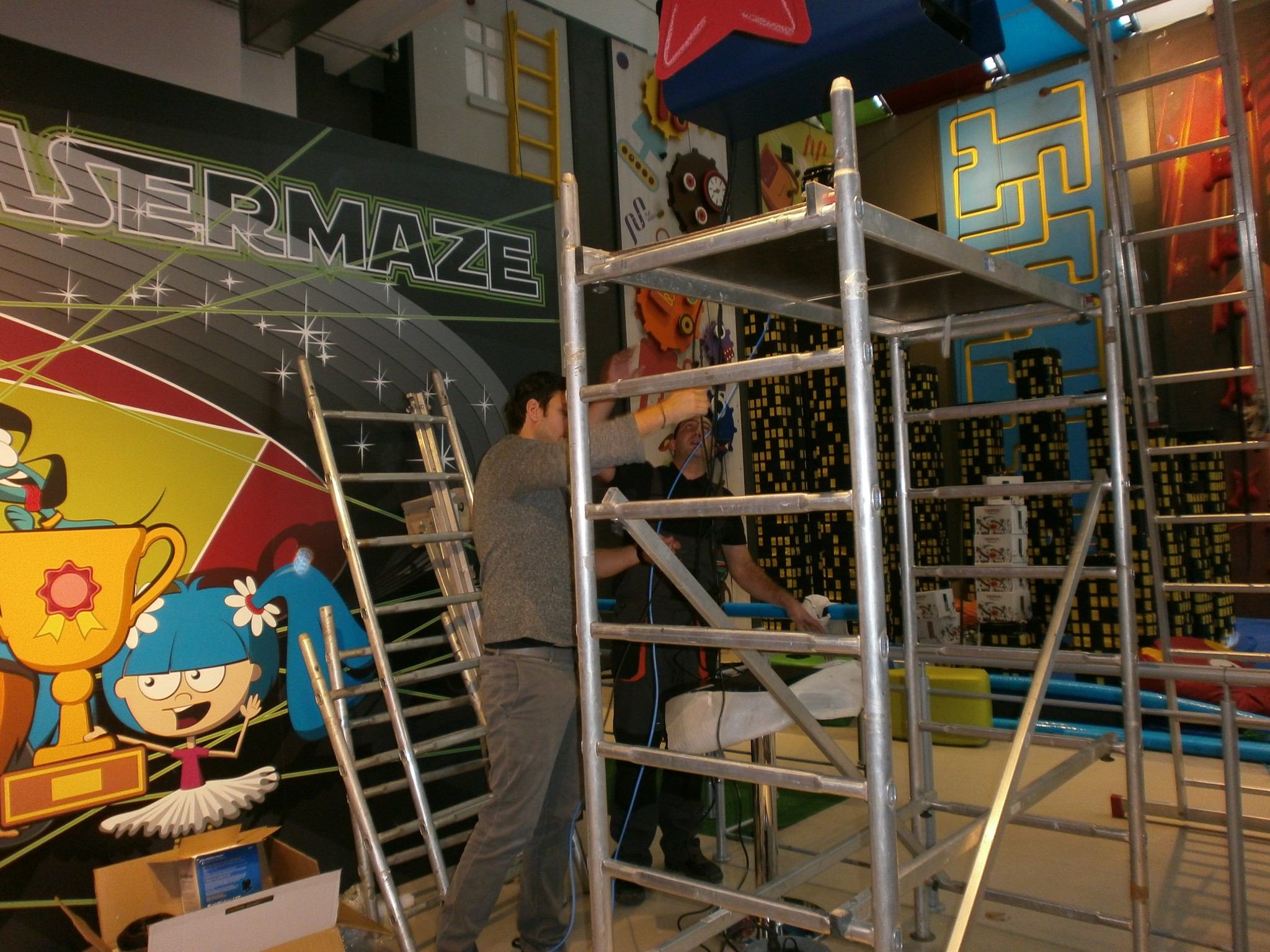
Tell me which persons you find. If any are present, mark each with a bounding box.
[585,346,824,914]
[429,374,714,952]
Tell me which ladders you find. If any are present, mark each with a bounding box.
[296,354,591,951]
[1100,28,1270,834]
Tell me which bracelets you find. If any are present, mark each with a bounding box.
[655,401,667,430]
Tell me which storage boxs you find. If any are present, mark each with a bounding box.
[973,499,1031,622]
[52,824,392,952]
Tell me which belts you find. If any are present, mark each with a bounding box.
[483,638,553,649]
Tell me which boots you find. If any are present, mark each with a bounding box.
[659,837,724,881]
[611,848,655,902]
